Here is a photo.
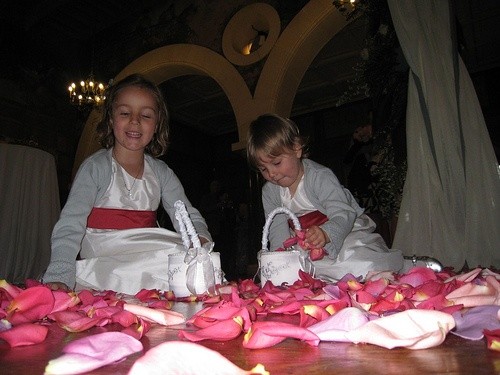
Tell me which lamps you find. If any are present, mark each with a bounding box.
[65,36,109,114]
[240,24,268,56]
[330,0,368,22]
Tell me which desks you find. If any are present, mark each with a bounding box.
[0,143,62,285]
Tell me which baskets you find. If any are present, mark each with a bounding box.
[167,200,221,298]
[259,206,315,288]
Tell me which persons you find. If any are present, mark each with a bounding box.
[37,75,229,300]
[245,114,442,282]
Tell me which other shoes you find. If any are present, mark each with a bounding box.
[402,254,443,273]
